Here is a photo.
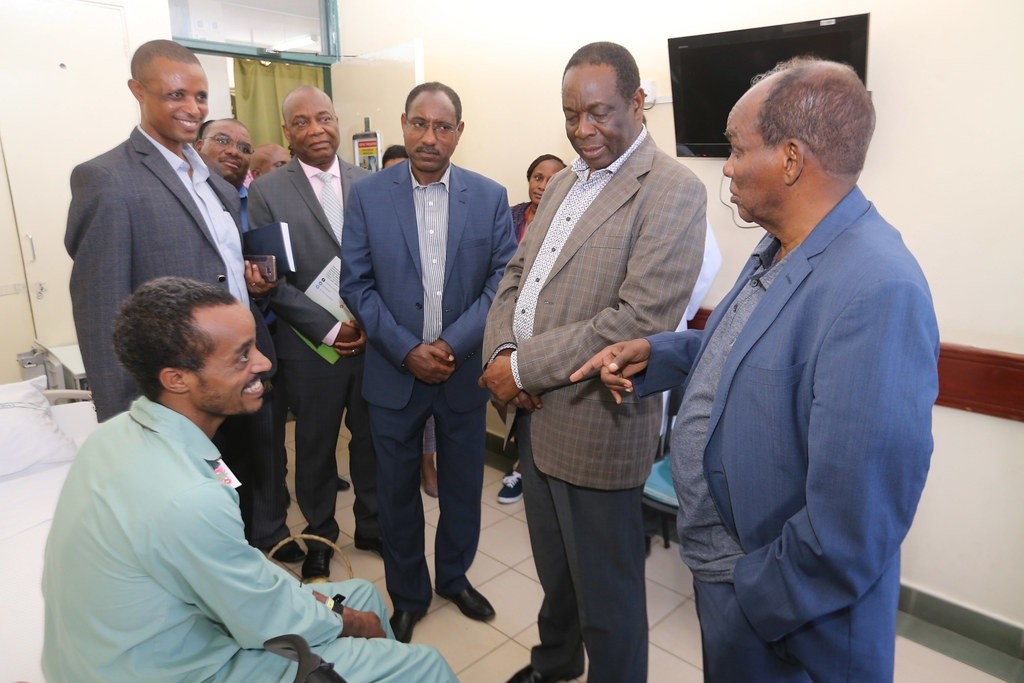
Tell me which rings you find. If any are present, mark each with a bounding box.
[352,350,357,356]
[252,283,255,286]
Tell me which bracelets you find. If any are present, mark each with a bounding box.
[326,597,334,610]
[331,593,346,615]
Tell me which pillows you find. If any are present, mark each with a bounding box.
[0,374,80,477]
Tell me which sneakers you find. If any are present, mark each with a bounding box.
[497,471,523,504]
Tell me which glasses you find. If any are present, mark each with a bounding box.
[208,135,254,154]
[407,119,459,134]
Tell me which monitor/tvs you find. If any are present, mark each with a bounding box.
[668,12,870,157]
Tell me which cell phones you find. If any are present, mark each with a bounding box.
[244,255,276,282]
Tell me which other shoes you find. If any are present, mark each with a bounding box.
[425,487,438,497]
[272,541,305,563]
[338,478,350,490]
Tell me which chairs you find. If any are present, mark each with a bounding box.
[642,387,679,548]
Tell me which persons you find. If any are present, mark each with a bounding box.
[63,40,437,585]
[478,42,722,683]
[570,54,939,683]
[40,276,458,683]
[496,153,567,504]
[338,82,518,645]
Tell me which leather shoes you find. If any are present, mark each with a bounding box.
[506,665,584,683]
[354,532,384,560]
[302,549,334,578]
[389,610,427,643]
[435,586,495,620]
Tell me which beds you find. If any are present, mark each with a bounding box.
[0,389,99,682]
